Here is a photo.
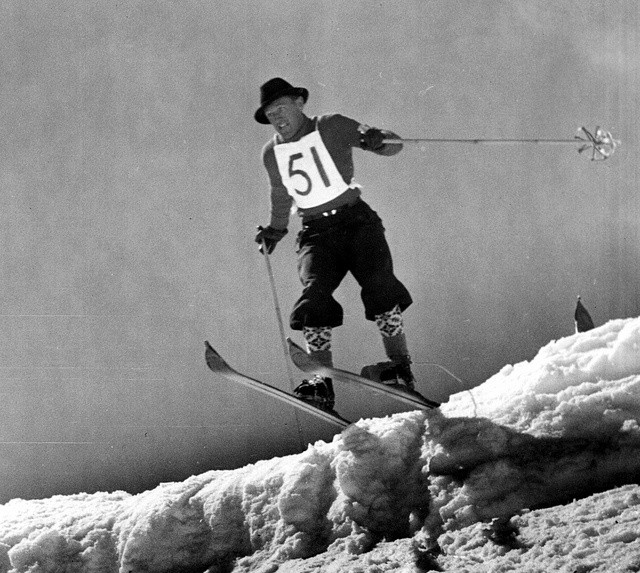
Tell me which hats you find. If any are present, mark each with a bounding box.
[253,77,309,125]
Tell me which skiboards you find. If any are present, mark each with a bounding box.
[202,339,441,431]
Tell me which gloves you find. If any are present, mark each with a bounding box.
[361,127,385,151]
[255,224,288,254]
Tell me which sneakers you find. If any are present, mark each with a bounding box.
[293,371,340,419]
[359,357,419,397]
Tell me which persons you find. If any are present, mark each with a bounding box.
[253,76,415,407]
